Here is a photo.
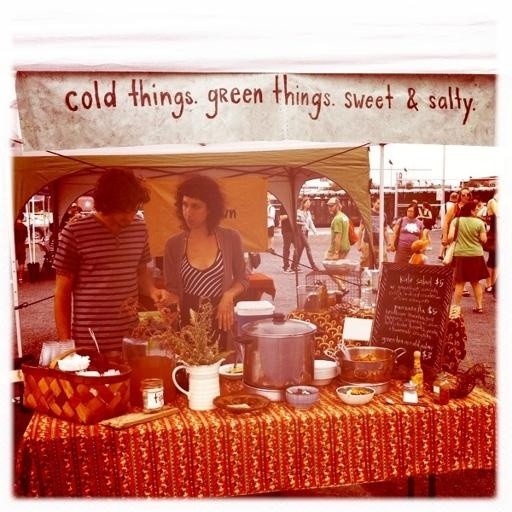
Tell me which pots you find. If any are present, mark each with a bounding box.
[324,344,407,384]
[232,313,318,388]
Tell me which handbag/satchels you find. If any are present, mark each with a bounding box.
[481,231,497,251]
[349,220,359,245]
[442,242,456,267]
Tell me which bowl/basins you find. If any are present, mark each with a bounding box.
[336,386,375,405]
[321,260,360,271]
[286,385,320,408]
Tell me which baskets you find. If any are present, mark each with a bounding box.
[21,347,133,426]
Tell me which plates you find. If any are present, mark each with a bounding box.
[313,360,337,387]
[219,363,243,381]
[212,393,272,412]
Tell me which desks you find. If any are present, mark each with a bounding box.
[14,373,496,497]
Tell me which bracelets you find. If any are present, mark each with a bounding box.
[333,249,340,256]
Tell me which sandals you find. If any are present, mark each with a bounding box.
[462,286,493,313]
[281,267,349,297]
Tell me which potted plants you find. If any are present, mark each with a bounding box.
[123,290,235,412]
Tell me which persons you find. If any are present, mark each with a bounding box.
[280,206,302,273]
[358,187,497,315]
[62,202,77,229]
[50,168,171,352]
[162,172,251,364]
[324,197,352,293]
[297,197,319,271]
[14,214,28,284]
[76,207,82,214]
[267,198,276,253]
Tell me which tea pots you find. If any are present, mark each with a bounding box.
[171,358,226,412]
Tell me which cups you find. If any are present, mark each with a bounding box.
[38,339,75,368]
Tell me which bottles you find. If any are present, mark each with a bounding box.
[402,351,450,405]
[140,379,165,414]
[360,267,373,308]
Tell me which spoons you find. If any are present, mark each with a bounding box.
[380,394,429,408]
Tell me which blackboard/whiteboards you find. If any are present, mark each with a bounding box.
[370,261,456,384]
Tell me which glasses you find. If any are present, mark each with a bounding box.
[460,193,470,198]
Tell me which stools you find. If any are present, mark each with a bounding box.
[241,273,276,301]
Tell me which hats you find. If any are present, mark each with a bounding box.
[327,197,339,205]
